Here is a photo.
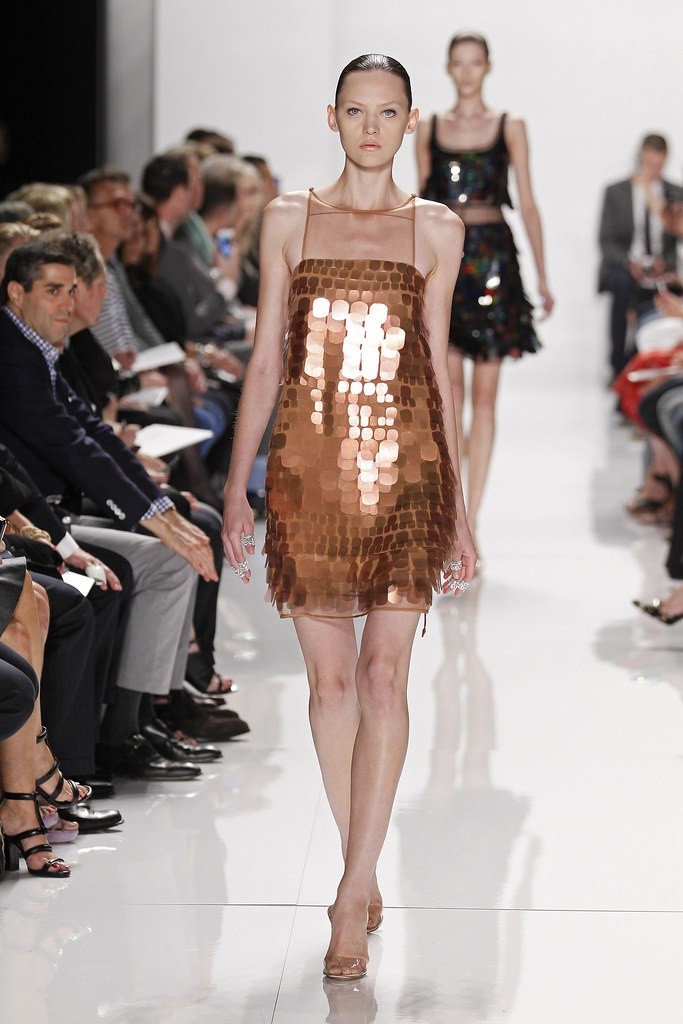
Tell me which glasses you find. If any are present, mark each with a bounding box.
[87,200,141,214]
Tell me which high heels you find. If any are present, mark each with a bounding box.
[36,726,92,809]
[1,791,70,877]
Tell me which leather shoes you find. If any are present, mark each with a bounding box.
[67,776,114,798]
[157,737,221,762]
[126,756,202,779]
[57,807,124,832]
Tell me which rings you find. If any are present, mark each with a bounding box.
[232,569,245,578]
[458,581,470,592]
[446,576,460,590]
[448,561,463,571]
[244,536,256,546]
[238,559,249,573]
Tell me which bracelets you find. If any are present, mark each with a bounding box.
[20,526,51,542]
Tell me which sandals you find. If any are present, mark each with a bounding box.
[322,904,369,980]
[367,894,382,933]
[186,640,233,694]
[39,804,79,843]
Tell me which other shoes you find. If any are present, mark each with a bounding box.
[153,690,250,744]
[627,472,676,526]
[635,600,683,625]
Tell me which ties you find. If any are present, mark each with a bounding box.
[644,207,651,256]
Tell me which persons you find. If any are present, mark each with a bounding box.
[416,33,556,565]
[1,126,279,880]
[220,54,477,980]
[595,135,683,625]
